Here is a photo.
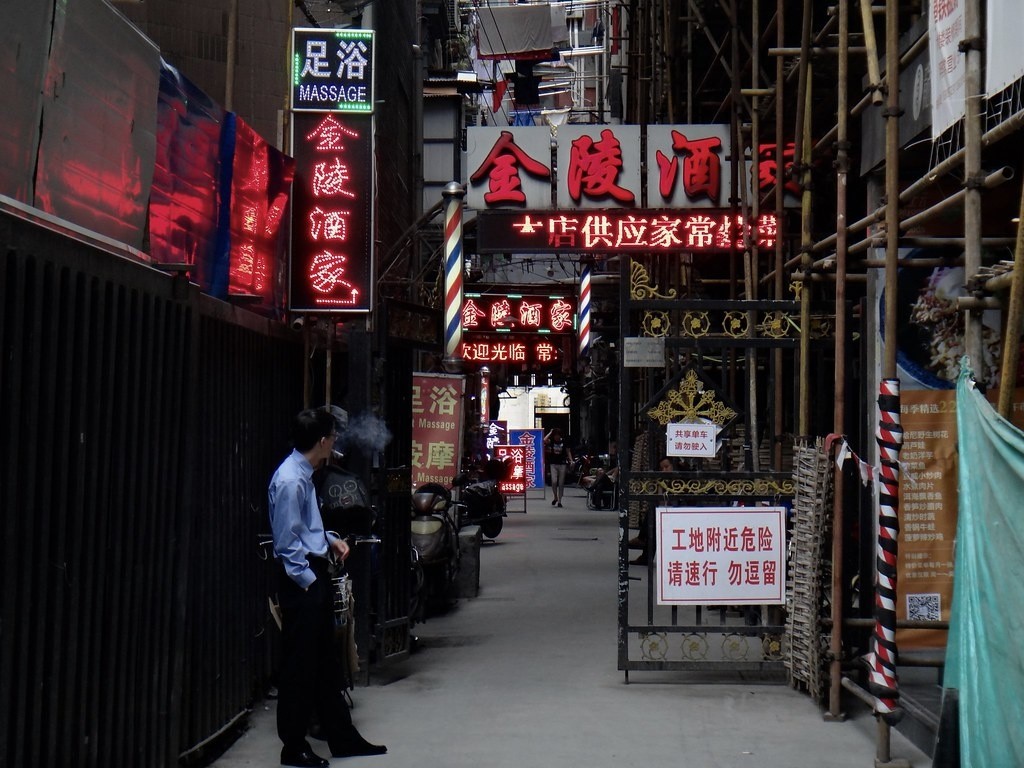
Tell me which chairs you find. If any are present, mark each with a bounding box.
[587,472,617,511]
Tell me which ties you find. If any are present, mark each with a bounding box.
[311,475,336,565]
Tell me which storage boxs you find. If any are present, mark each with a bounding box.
[486,455,514,481]
[415,480,451,514]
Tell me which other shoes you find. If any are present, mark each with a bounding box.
[558,503,562,507]
[629,554,649,566]
[552,499,558,505]
[584,487,594,494]
[629,537,645,549]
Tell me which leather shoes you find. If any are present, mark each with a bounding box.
[280,743,329,768]
[328,729,387,757]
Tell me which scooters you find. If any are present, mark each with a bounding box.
[411,455,515,621]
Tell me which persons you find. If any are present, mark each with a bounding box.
[627,456,687,566]
[584,440,617,510]
[269,404,388,768]
[543,427,575,508]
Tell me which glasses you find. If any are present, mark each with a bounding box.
[320,433,341,441]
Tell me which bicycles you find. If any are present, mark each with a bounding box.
[258,530,381,710]
